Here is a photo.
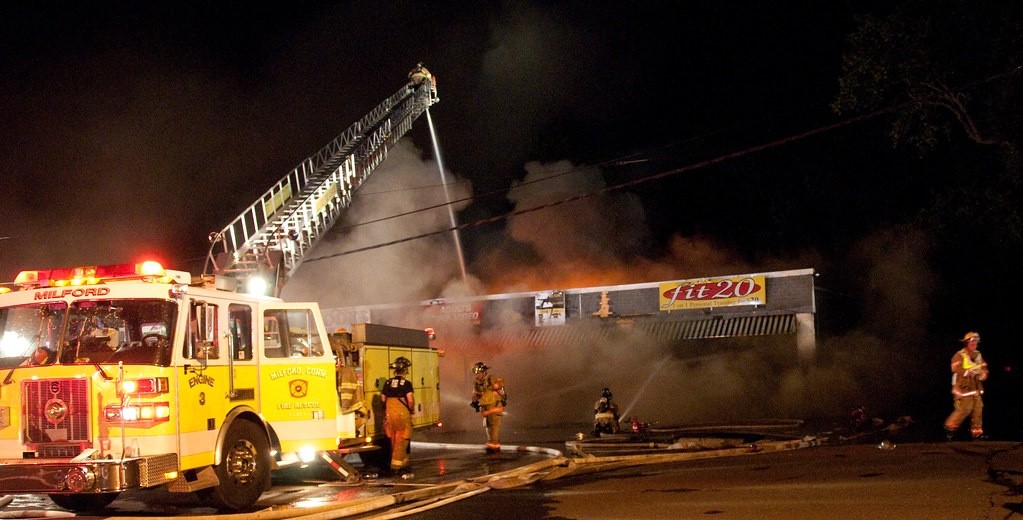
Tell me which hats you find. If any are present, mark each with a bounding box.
[390,358,410,372]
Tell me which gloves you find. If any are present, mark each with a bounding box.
[472,401,480,413]
[501,398,506,407]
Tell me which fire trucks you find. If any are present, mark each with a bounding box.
[0,63,443,514]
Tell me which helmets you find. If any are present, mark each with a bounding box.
[961,332,980,343]
[600,396,607,402]
[600,388,613,397]
[472,361,489,375]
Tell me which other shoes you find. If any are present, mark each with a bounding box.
[486,446,500,453]
[392,465,413,476]
[946,429,959,444]
[971,435,987,441]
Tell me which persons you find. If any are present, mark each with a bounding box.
[943,332,994,442]
[377,356,416,478]
[469,362,508,454]
[592,388,621,438]
[53,313,120,353]
